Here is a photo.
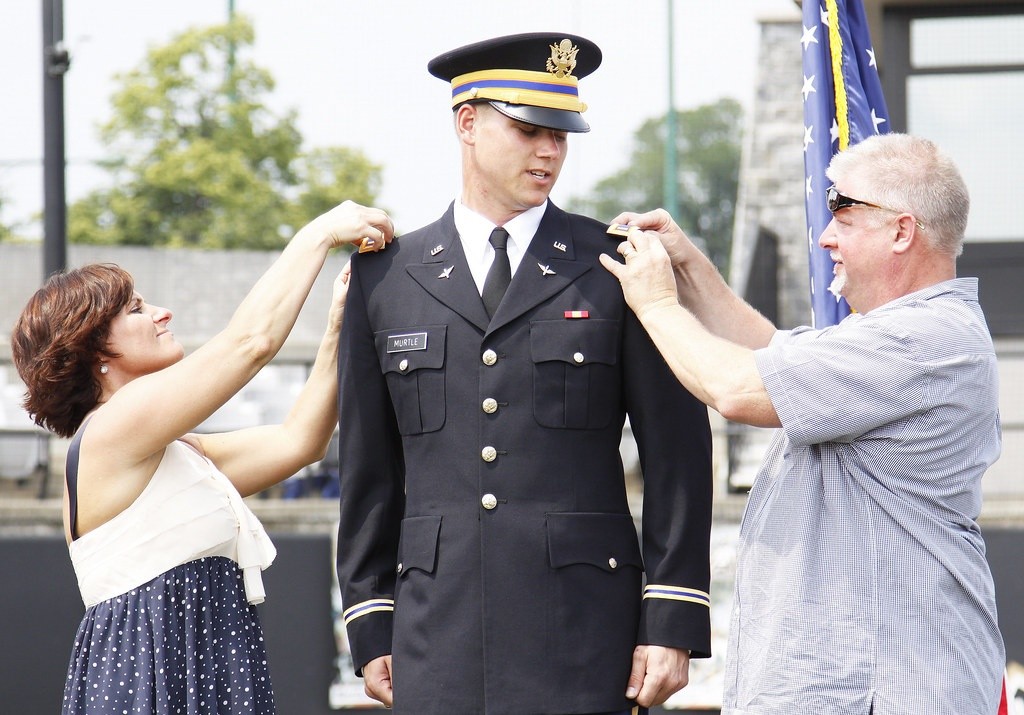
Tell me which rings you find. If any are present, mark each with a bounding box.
[623,247,636,257]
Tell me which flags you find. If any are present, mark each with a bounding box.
[802,1,891,331]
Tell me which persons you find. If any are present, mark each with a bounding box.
[600,133,1006,715]
[13,198,395,715]
[336,31,712,715]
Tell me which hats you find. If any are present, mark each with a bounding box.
[427,31,602,133]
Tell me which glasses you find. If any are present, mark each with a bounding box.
[825,184,924,230]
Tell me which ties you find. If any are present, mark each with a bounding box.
[481,227,511,322]
